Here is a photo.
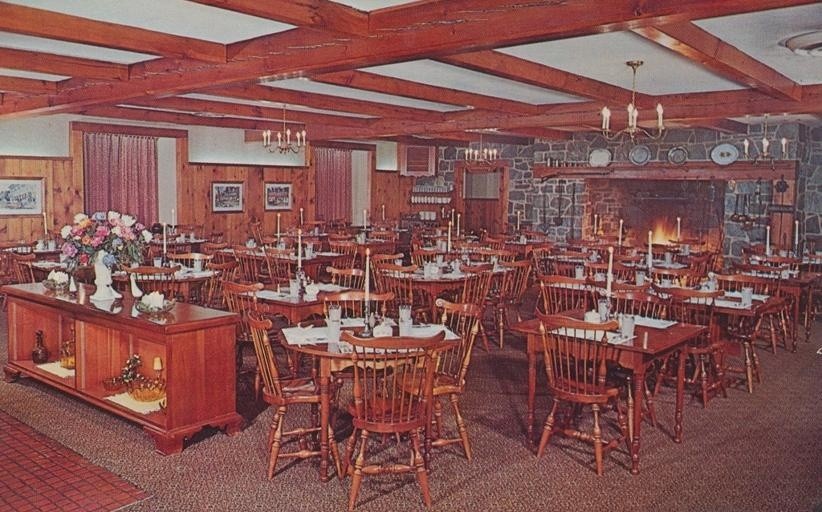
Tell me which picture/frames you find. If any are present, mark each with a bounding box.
[264,181,294,211]
[0,176,46,218]
[210,181,244,213]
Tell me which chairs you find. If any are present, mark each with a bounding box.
[0,219,821,512]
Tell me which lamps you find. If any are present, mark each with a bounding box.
[744,114,786,160]
[262,105,307,153]
[603,59,662,141]
[466,129,497,165]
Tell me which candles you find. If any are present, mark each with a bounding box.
[594,215,597,235]
[457,214,460,236]
[364,209,366,229]
[517,211,520,230]
[277,213,280,240]
[300,208,303,225]
[451,209,454,226]
[172,209,174,228]
[43,212,47,234]
[382,205,385,221]
